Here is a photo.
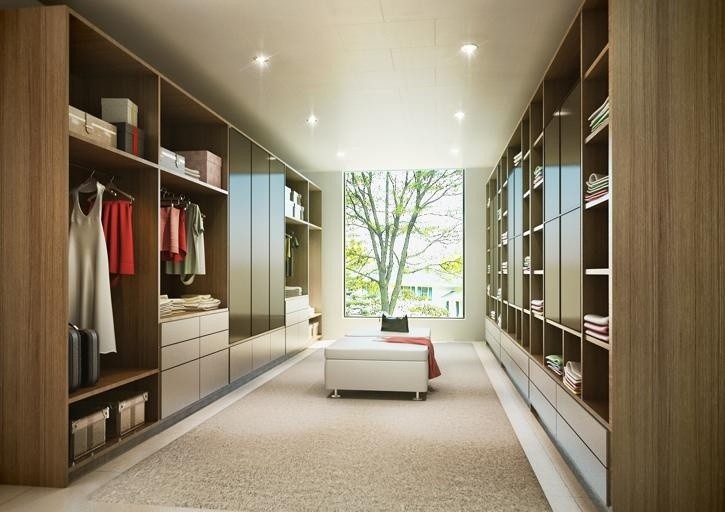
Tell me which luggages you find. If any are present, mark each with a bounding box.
[68,321,100,393]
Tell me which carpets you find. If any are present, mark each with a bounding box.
[85,340,553,511]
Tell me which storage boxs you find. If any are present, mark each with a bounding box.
[176,148,222,186]
[71,407,108,462]
[100,97,139,126]
[310,321,320,338]
[112,388,149,439]
[285,186,305,219]
[68,105,118,148]
[110,121,147,160]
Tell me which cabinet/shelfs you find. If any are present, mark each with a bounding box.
[485,154,501,362]
[529,0,613,505]
[228,123,323,384]
[0,4,229,488]
[500,102,529,405]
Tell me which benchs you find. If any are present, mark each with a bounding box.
[324,326,430,401]
